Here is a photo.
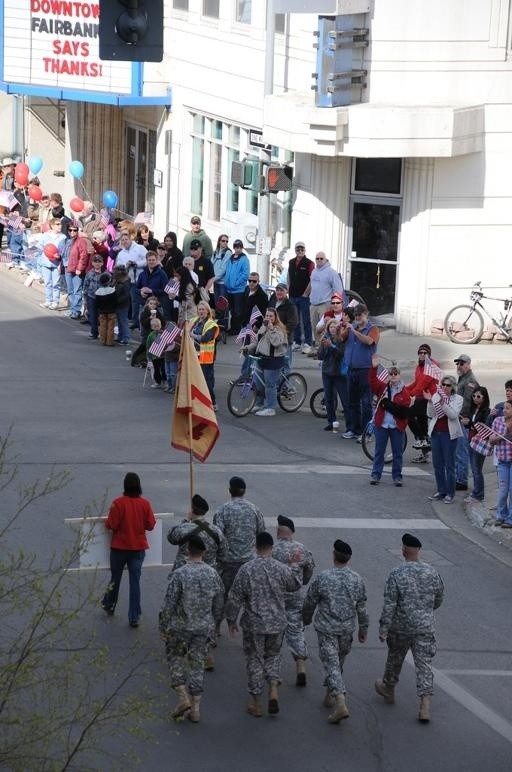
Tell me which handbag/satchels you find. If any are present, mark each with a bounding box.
[381,384,410,419]
[470,434,493,456]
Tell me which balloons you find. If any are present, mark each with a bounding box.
[70,198,84,211]
[29,185,41,201]
[45,243,58,261]
[69,160,83,179]
[15,163,29,186]
[29,156,42,175]
[103,191,117,208]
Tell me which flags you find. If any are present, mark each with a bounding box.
[100,207,110,227]
[172,320,220,463]
[0,250,12,263]
[475,421,493,440]
[8,214,23,229]
[250,305,263,325]
[160,322,179,344]
[236,324,257,345]
[377,364,390,385]
[5,194,18,210]
[164,278,180,297]
[148,334,165,357]
[135,211,152,223]
[433,385,449,419]
[423,355,443,382]
[0,216,8,227]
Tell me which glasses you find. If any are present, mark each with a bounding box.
[456,363,467,366]
[473,394,484,399]
[442,383,452,388]
[316,257,323,260]
[68,229,78,232]
[295,249,307,251]
[331,301,341,304]
[248,279,258,283]
[419,351,429,354]
[389,373,398,376]
[222,239,229,242]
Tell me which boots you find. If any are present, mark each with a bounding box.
[171,686,190,718]
[246,695,262,717]
[190,694,201,721]
[205,652,215,670]
[268,682,279,714]
[419,696,430,721]
[323,688,333,707]
[375,680,395,702]
[296,659,306,686]
[329,695,350,724]
[215,624,221,635]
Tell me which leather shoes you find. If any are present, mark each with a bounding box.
[101,603,114,615]
[455,483,468,490]
[130,619,139,627]
[308,349,318,357]
[323,424,332,430]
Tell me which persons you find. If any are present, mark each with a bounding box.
[39,194,70,227]
[423,377,463,504]
[158,322,181,394]
[145,318,166,389]
[185,300,218,413]
[453,355,479,490]
[156,243,173,277]
[136,252,168,344]
[182,216,212,259]
[161,232,184,278]
[231,321,260,385]
[82,231,108,321]
[102,473,157,627]
[93,273,116,345]
[315,293,348,341]
[168,495,225,671]
[460,387,490,505]
[318,319,349,435]
[37,219,65,312]
[212,475,266,640]
[267,283,298,397]
[115,234,147,331]
[240,272,267,331]
[80,203,133,249]
[135,226,160,251]
[213,235,231,332]
[487,380,512,510]
[303,541,369,724]
[82,256,111,340]
[160,538,222,723]
[54,224,87,319]
[405,345,439,463]
[340,303,380,443]
[240,306,287,417]
[343,306,355,323]
[224,240,250,337]
[489,401,512,528]
[307,251,343,359]
[141,297,162,368]
[268,516,316,682]
[184,257,198,286]
[1,158,14,198]
[189,240,214,302]
[12,231,43,287]
[173,267,199,329]
[113,266,130,344]
[376,532,443,722]
[368,353,412,487]
[287,242,315,354]
[226,533,300,718]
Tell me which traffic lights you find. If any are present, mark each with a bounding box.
[265,165,293,191]
[98,0,163,61]
[230,160,253,185]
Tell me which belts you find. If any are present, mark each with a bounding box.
[313,301,330,307]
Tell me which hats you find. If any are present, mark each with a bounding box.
[278,515,295,532]
[418,344,431,353]
[190,239,200,248]
[296,241,305,247]
[192,494,209,510]
[0,158,17,166]
[229,476,246,488]
[334,539,353,554]
[191,216,200,222]
[332,292,343,300]
[402,534,421,547]
[352,305,368,315]
[158,243,166,249]
[92,255,103,261]
[100,272,111,285]
[454,354,471,362]
[258,532,274,544]
[275,283,288,291]
[189,534,206,550]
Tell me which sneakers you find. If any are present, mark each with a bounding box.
[164,387,171,393]
[120,341,128,345]
[71,313,80,318]
[464,497,482,503]
[469,493,474,497]
[393,478,402,486]
[169,389,177,393]
[292,344,300,351]
[255,409,274,416]
[495,521,503,526]
[357,436,371,443]
[5,262,17,268]
[411,454,431,462]
[249,406,262,412]
[49,304,58,309]
[115,339,121,342]
[302,345,312,353]
[163,381,166,386]
[40,302,50,308]
[88,334,96,339]
[65,310,72,317]
[428,494,445,500]
[444,495,454,504]
[412,440,426,448]
[370,477,379,485]
[151,383,160,388]
[342,432,360,439]
[502,522,512,528]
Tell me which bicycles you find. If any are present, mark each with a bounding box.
[444,284,512,344]
[228,349,308,418]
[363,404,438,466]
[311,389,339,418]
